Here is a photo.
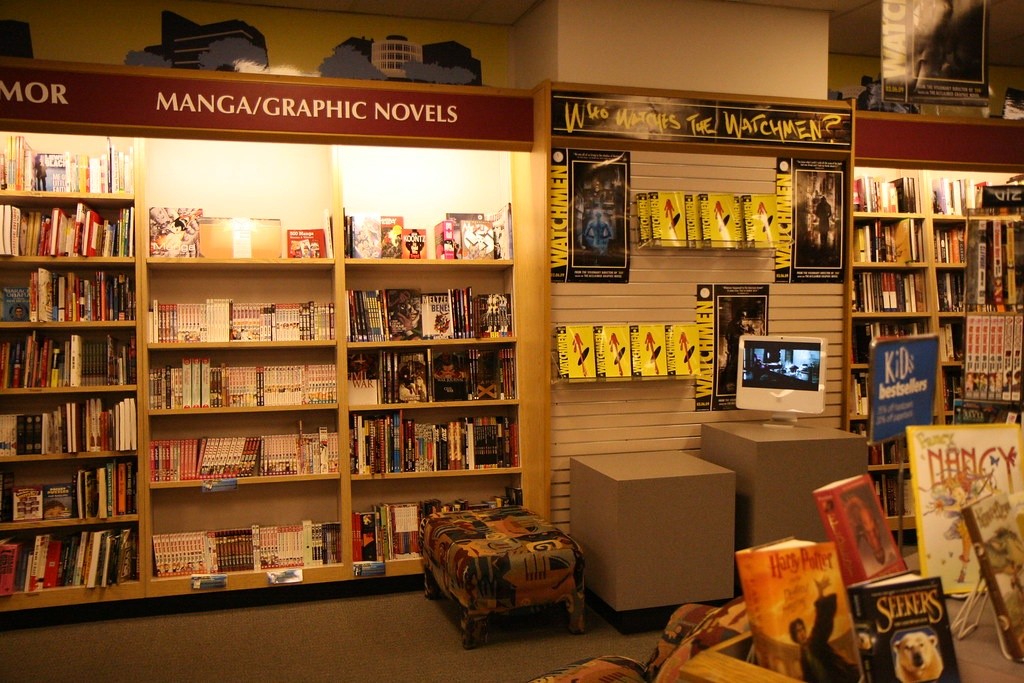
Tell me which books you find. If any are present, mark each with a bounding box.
[148,207,343,579]
[846,569,960,683]
[637,191,780,249]
[555,320,701,380]
[345,202,523,561]
[849,173,1023,516]
[0,130,139,598]
[735,534,865,683]
[812,473,909,587]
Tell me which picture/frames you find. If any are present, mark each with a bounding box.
[949,507,1024,665]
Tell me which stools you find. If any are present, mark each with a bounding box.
[418,506,586,650]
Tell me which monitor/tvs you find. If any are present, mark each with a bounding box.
[735,335,826,427]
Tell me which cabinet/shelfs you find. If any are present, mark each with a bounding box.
[1,81,552,611]
[551,239,794,383]
[848,166,1024,530]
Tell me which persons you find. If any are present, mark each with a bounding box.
[815,196,831,248]
[718,307,756,382]
